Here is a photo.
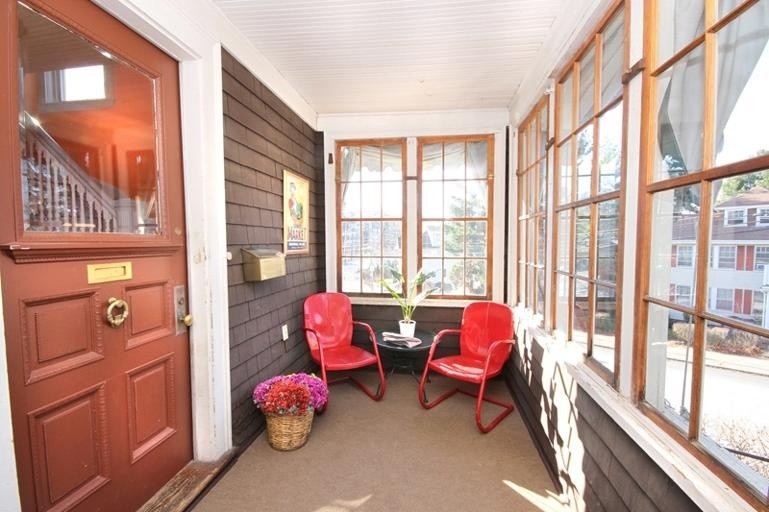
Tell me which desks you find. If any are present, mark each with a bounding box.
[368,327,434,402]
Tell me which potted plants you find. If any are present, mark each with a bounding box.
[377,267,438,337]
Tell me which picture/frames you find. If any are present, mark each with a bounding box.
[283,169,310,256]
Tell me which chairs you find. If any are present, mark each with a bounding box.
[301,292,386,415]
[418,302,516,434]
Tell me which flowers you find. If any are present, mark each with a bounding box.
[253,372,329,415]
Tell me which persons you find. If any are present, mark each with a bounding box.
[289,182,300,227]
